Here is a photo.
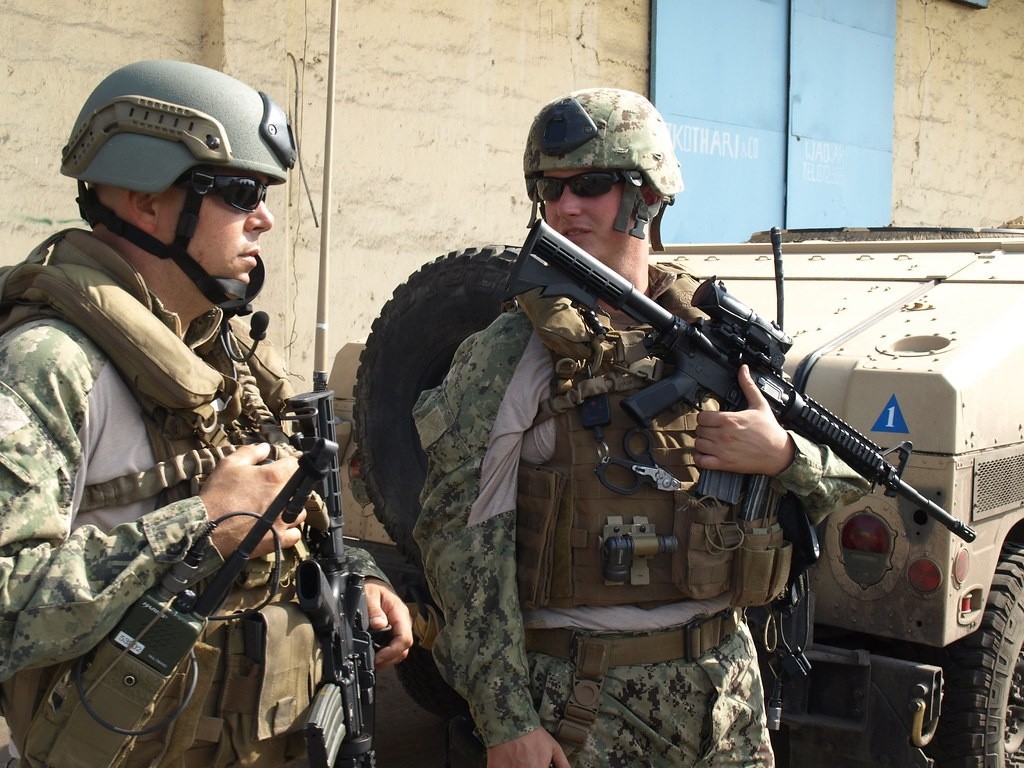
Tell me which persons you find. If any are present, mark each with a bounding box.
[411,88,872,768]
[0,59,415,768]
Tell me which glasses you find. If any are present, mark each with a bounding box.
[533,172,626,202]
[171,172,269,213]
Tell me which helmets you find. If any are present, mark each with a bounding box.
[524,87,682,204]
[60,60,297,194]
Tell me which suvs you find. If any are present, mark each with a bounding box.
[324,214,1023,768]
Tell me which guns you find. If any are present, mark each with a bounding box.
[285,372,372,768]
[507,217,975,542]
